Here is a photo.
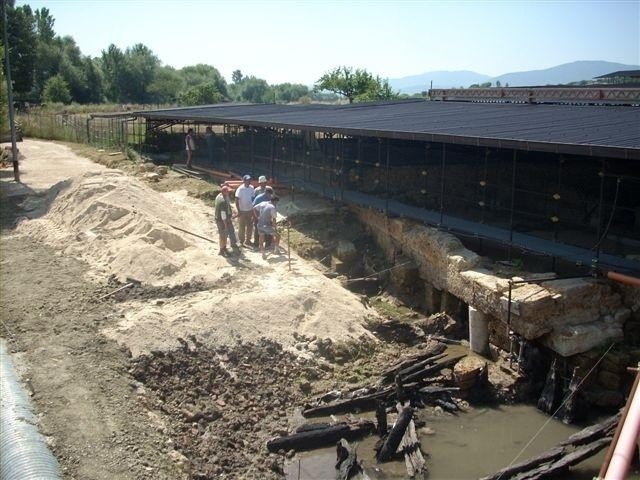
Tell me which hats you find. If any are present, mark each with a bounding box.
[257,175,267,184]
[221,185,233,193]
[242,174,252,183]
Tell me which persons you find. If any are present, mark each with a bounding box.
[234,175,254,247]
[215,183,238,248]
[254,176,273,198]
[200,126,218,166]
[252,197,281,259]
[214,185,229,257]
[252,188,272,250]
[185,128,195,169]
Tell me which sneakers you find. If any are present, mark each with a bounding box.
[232,240,279,251]
[219,247,231,258]
